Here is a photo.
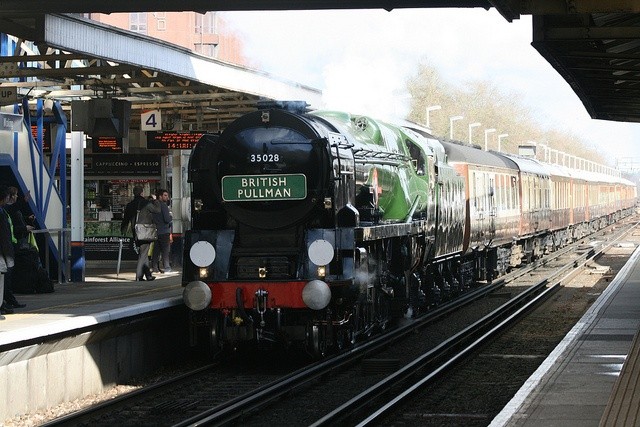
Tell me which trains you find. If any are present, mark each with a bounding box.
[170,100,639,375]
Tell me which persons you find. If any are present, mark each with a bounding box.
[120,185,158,282]
[147,192,155,198]
[149,188,177,273]
[9,186,34,308]
[0,186,15,315]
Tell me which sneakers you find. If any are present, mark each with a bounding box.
[1,304,13,314]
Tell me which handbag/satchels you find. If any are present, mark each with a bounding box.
[134,197,159,242]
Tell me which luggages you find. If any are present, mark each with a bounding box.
[12,230,38,294]
